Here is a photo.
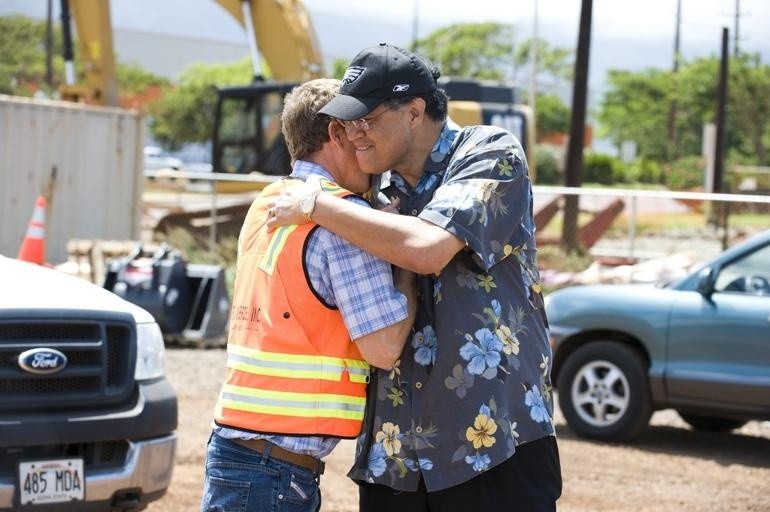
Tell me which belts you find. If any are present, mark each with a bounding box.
[227,432,326,479]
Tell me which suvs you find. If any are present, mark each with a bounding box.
[0,250,199,510]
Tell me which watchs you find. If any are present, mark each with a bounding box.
[297,188,319,223]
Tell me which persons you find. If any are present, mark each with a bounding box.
[260,42,563,512]
[202,77,418,511]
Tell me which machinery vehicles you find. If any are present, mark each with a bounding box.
[48,1,612,273]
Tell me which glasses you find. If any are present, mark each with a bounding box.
[335,97,411,131]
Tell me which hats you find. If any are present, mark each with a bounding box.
[316,41,438,121]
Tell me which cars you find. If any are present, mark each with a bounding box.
[534,227,769,432]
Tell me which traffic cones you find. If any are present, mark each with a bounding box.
[15,195,53,266]
